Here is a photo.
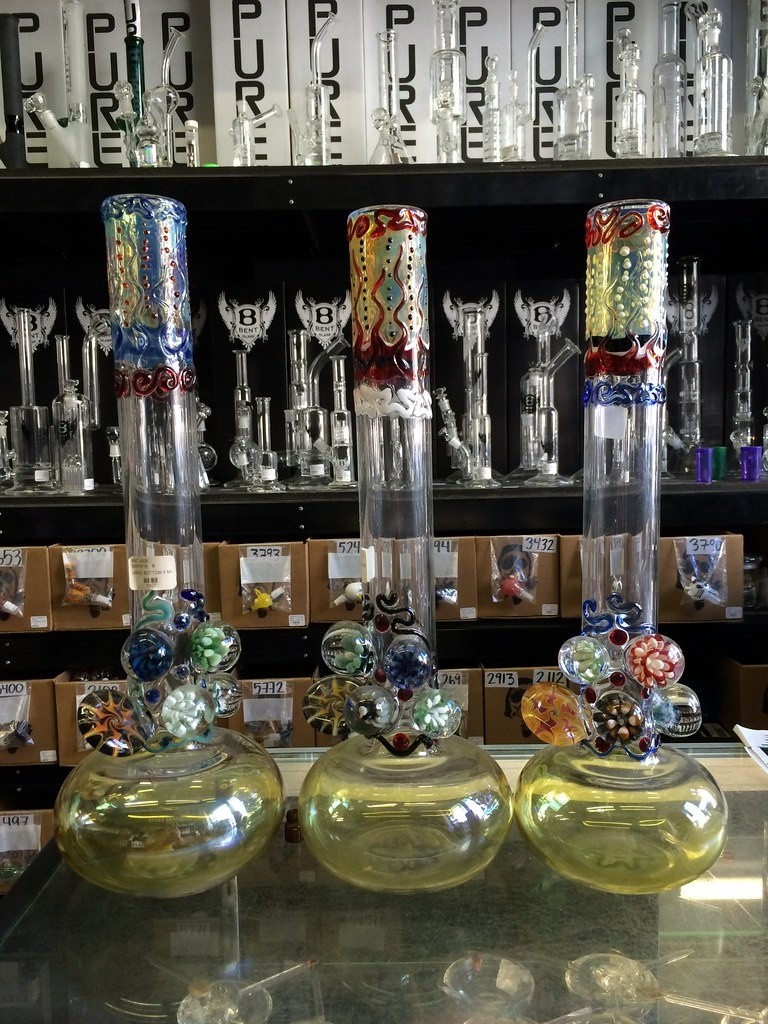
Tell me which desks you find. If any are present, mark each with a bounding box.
[0,743,768,1024]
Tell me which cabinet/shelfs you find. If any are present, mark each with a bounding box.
[0,13,767,786]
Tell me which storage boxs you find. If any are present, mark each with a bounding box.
[2,531,767,767]
[0,0,767,164]
[0,809,55,896]
